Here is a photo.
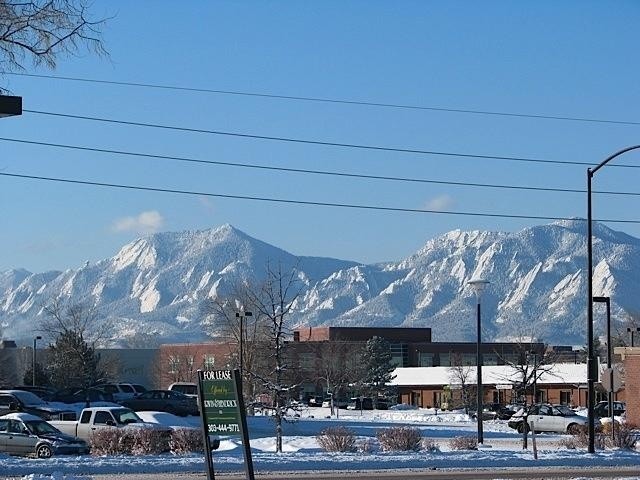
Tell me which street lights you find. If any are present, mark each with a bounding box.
[33,335,42,387]
[236,311,253,393]
[593,297,612,417]
[627,327,640,347]
[466,279,491,443]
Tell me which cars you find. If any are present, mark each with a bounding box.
[468,401,626,435]
[0,378,220,459]
[308,394,389,411]
[441,398,464,411]
